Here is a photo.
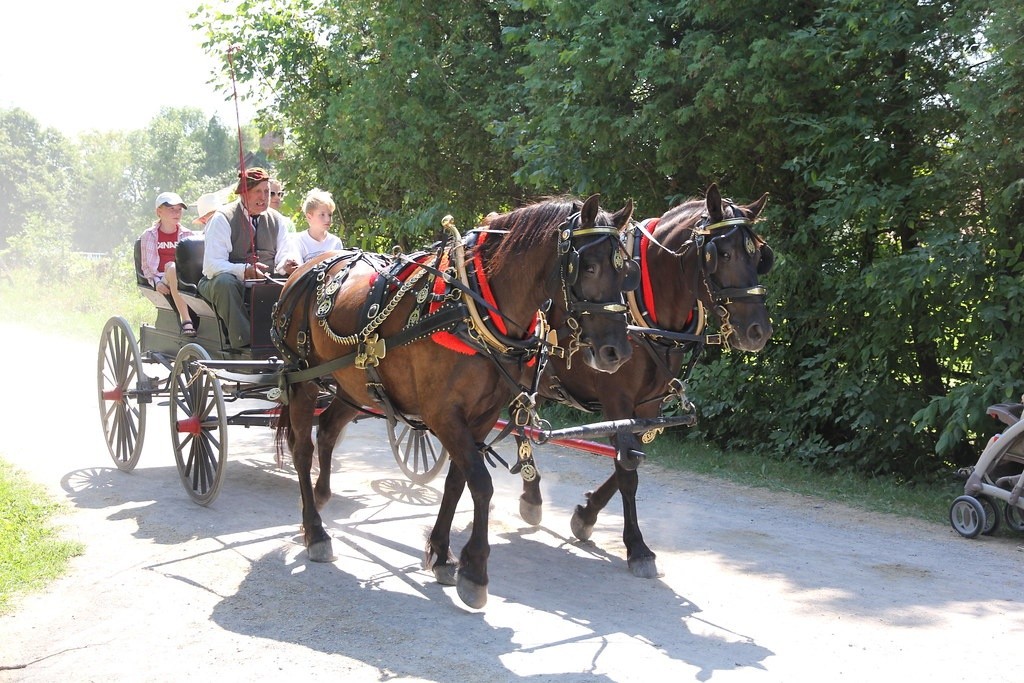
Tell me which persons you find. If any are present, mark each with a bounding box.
[269,179,297,233]
[296,187,344,263]
[140,192,197,337]
[192,193,223,225]
[198,167,304,348]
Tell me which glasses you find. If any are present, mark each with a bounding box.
[271,192,284,197]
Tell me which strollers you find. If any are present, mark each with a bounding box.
[948,394,1023,539]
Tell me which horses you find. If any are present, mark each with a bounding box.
[268,181,773,610]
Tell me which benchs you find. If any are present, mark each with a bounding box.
[133,238,175,309]
[173,234,218,317]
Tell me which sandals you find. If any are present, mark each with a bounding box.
[181,322,196,337]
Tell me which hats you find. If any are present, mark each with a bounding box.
[192,194,225,224]
[156,192,187,210]
[235,167,269,195]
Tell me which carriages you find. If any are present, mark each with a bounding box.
[95,181,775,611]
